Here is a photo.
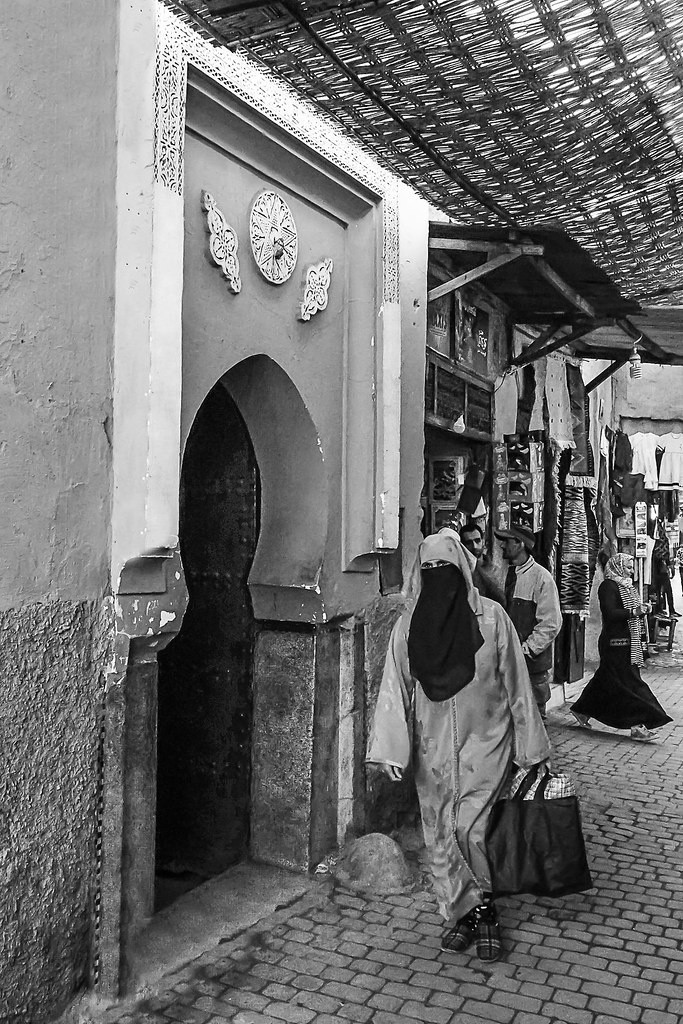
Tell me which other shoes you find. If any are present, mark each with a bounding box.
[474,904,502,963]
[571,709,591,730]
[440,914,475,954]
[631,725,659,741]
[669,612,682,618]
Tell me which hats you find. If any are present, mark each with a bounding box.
[494,524,536,550]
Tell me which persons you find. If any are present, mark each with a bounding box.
[495,525,563,722]
[655,552,683,618]
[569,553,674,743]
[366,534,552,963]
[459,525,507,608]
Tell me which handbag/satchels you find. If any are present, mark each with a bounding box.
[487,763,593,898]
